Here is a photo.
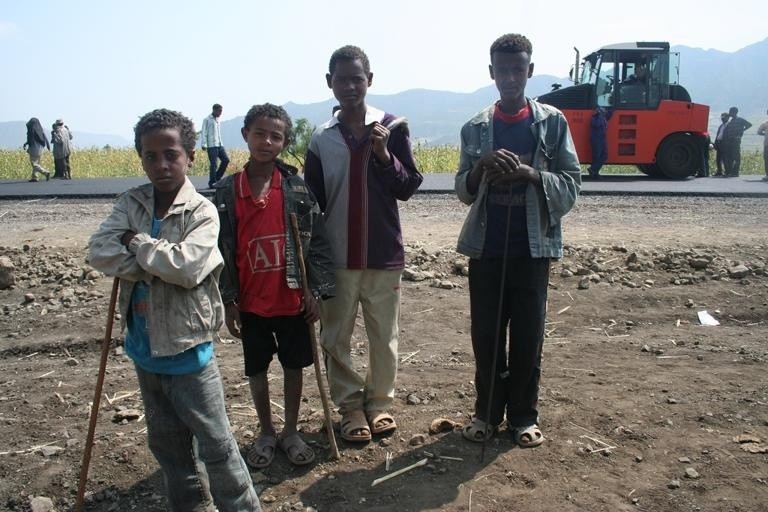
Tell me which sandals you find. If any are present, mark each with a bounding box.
[462,417,496,442]
[247,432,276,468]
[367,408,396,434]
[341,412,371,441]
[278,433,315,465]
[511,420,544,447]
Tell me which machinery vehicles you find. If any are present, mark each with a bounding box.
[532,41,713,180]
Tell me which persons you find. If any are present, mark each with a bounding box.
[23,118,52,183]
[54,120,70,179]
[50,123,56,178]
[587,107,617,179]
[83,108,263,512]
[724,108,751,177]
[713,113,730,176]
[64,125,73,177]
[757,110,768,181]
[455,35,582,447]
[302,46,424,442]
[212,104,332,470]
[200,104,230,189]
